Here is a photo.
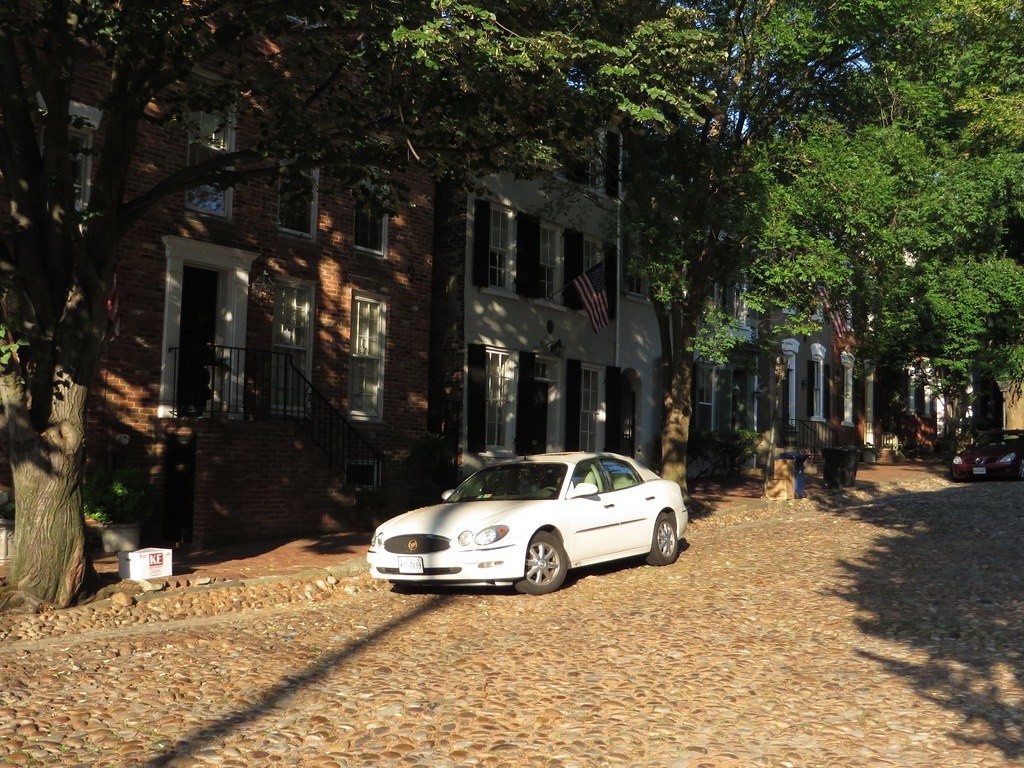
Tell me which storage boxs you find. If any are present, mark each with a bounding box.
[117,547,173,579]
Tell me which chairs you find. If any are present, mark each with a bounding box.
[574,469,596,485]
[519,473,541,494]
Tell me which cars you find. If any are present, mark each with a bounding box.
[367,451,690,598]
[949,429,1024,483]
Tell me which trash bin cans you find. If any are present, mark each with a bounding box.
[820,446,863,489]
[780,452,810,498]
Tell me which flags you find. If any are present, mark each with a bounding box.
[817,284,853,338]
[571,257,609,334]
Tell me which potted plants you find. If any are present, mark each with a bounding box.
[81,464,158,552]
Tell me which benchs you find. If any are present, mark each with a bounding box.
[609,470,636,489]
